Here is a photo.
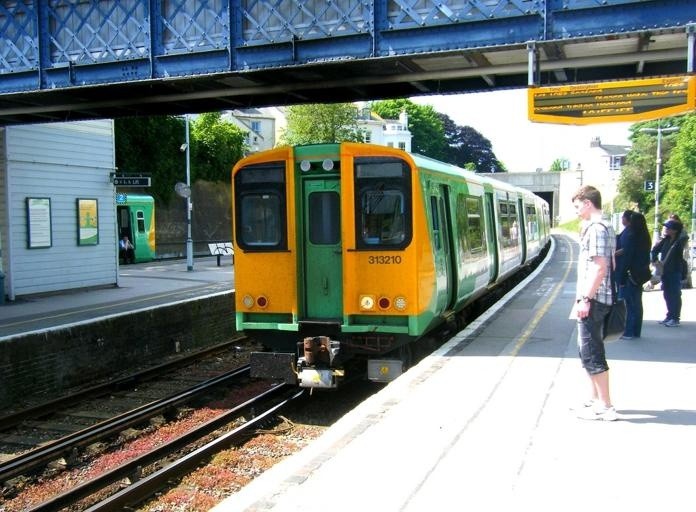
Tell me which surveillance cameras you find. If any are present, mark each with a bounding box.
[180,143,187,152]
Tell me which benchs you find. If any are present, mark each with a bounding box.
[208,242,234,266]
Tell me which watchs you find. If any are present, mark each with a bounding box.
[581,294,594,303]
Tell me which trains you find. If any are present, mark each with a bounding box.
[231,135,555,391]
[116,191,157,265]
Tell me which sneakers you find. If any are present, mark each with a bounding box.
[571,401,617,420]
[658,316,680,326]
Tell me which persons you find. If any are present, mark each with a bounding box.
[119,234,135,264]
[615,209,690,340]
[569,185,622,422]
[526,216,535,241]
[511,222,518,247]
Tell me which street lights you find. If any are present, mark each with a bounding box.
[638,125,681,248]
[602,151,627,227]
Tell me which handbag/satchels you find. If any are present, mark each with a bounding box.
[626,264,652,286]
[603,297,626,342]
[683,260,687,279]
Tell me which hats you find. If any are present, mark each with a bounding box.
[661,221,681,230]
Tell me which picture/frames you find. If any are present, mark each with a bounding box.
[76,198,99,245]
[26,197,52,248]
